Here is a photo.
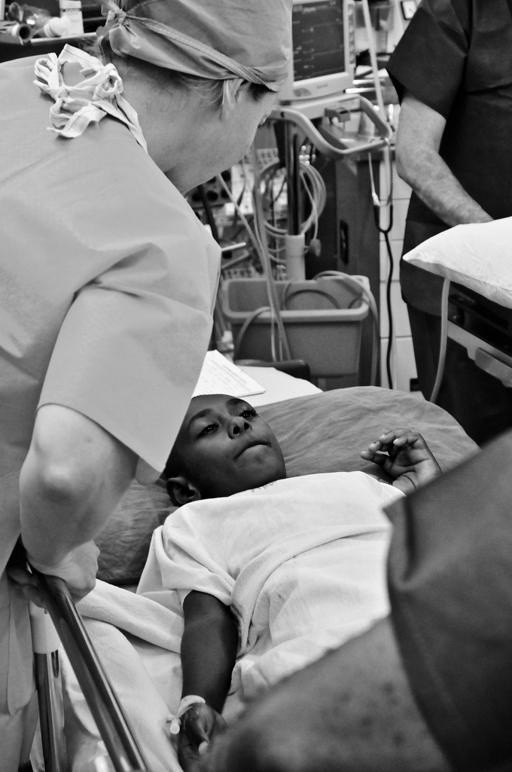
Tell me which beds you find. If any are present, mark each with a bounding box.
[3,345,510,765]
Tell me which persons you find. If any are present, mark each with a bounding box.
[165,392,442,765]
[0,0,297,772]
[395,1,512,450]
[201,431,512,766]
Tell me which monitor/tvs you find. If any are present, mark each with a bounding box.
[275,0,356,101]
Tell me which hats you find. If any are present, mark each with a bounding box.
[99,0,295,96]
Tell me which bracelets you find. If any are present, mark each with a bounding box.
[165,690,209,733]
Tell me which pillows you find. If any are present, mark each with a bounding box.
[95,384,486,584]
[399,212,511,312]
